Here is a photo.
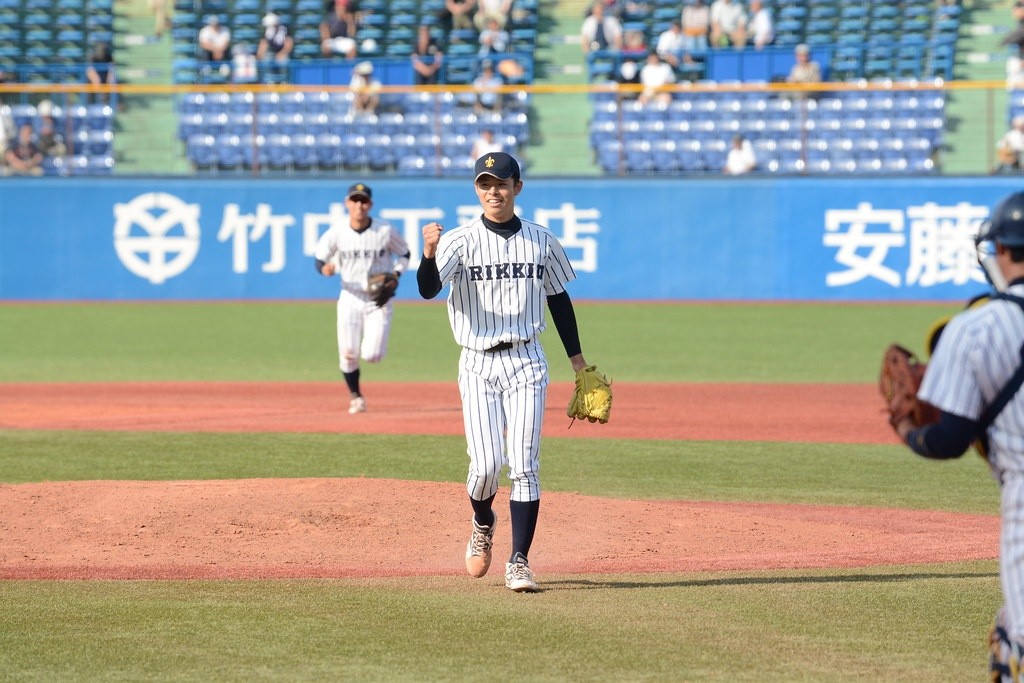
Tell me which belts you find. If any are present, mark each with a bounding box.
[486,339,530,353]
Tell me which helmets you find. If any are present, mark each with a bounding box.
[983,190,1024,245]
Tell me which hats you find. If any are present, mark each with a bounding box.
[474,151,521,181]
[348,184,372,201]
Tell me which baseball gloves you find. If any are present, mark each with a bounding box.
[567,364,613,424]
[368,272,401,307]
[878,344,938,429]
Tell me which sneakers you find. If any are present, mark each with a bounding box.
[348,396,365,414]
[505,551,537,590]
[465,507,497,578]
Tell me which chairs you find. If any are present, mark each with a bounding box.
[170,0,541,179]
[0,0,118,176]
[584,0,964,180]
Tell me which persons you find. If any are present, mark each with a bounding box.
[314,183,411,415]
[319,0,356,59]
[440,0,523,169]
[0,42,115,174]
[788,44,823,101]
[149,0,178,41]
[997,0,1024,169]
[579,0,776,84]
[412,25,442,86]
[639,51,676,111]
[256,12,294,84]
[725,135,756,176]
[229,43,259,83]
[418,152,613,591]
[350,60,380,115]
[880,191,1023,682]
[199,13,231,75]
